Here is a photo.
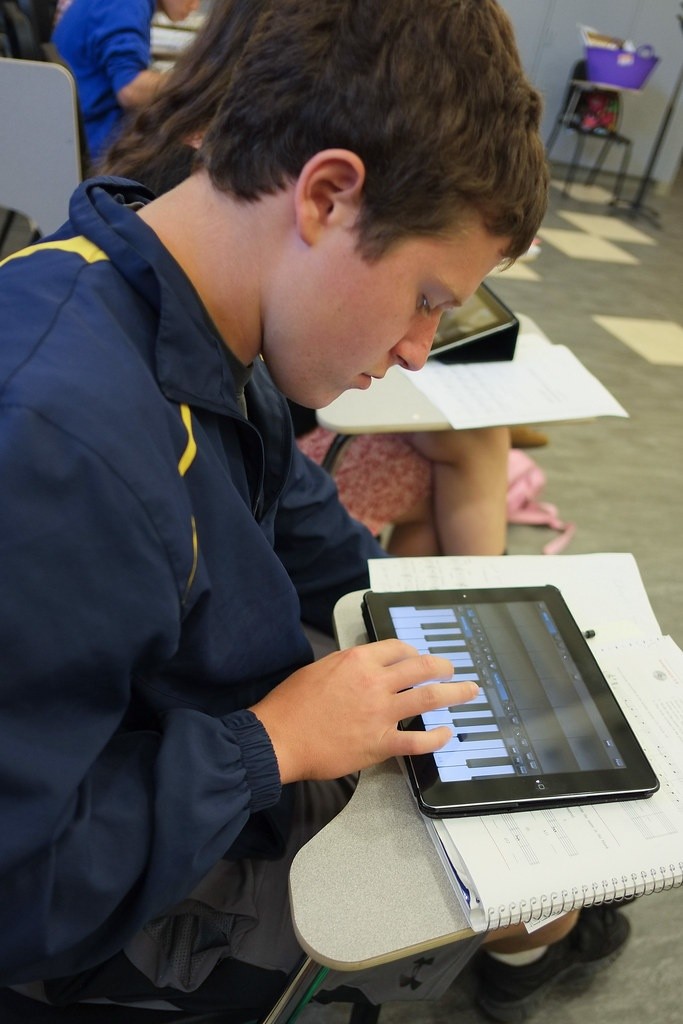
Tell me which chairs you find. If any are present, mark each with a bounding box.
[0,51,84,257]
[544,57,634,206]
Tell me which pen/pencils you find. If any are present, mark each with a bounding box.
[582,630,595,639]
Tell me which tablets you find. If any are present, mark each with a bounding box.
[363,585,660,814]
[427,283,516,356]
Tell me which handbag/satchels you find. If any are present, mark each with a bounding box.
[507,443,575,555]
[291,420,436,536]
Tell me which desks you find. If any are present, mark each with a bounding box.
[256,578,679,1023]
[317,310,599,506]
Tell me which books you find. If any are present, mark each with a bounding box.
[367,552,682,935]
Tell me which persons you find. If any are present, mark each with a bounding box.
[0,0,635,1024]
[51,0,203,182]
[95,0,508,556]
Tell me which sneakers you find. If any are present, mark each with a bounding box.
[473,905,630,1023]
